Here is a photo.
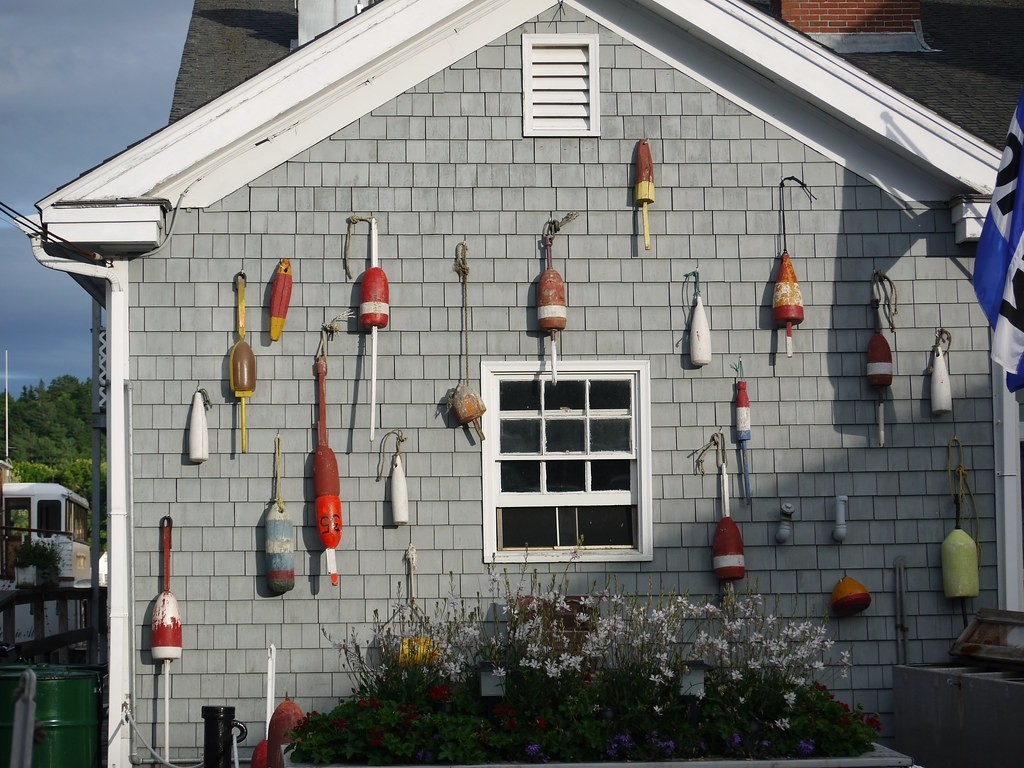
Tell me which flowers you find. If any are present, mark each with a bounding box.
[284,565,884,763]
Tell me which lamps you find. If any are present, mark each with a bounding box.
[673,659,716,698]
[470,661,514,698]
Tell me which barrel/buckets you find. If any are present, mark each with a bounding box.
[0,664,103,768]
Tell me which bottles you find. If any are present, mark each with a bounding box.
[391,455,409,524]
[931,346,952,413]
[941,525,979,598]
[690,297,712,366]
[189,392,210,461]
[266,498,295,592]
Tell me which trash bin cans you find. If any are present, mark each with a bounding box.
[0,663,109,768]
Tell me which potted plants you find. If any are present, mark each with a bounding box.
[14,546,65,589]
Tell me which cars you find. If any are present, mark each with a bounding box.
[97,552,108,587]
